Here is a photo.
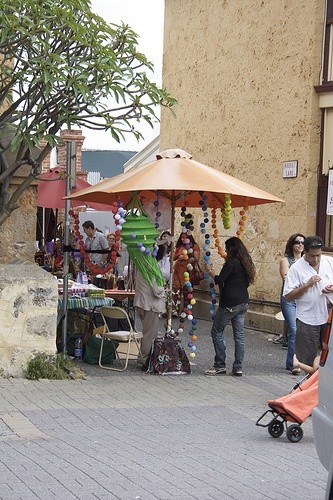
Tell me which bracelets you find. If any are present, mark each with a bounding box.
[208,270,212,274]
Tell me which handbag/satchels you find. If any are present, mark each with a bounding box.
[84,336,120,364]
[189,257,204,281]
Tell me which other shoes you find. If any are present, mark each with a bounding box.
[292,370,300,375]
[283,343,288,349]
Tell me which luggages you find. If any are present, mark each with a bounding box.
[257,367,319,442]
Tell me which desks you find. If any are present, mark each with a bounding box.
[56,297,114,332]
[106,290,136,296]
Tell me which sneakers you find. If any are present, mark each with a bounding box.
[273,334,289,344]
[231,370,242,376]
[205,366,226,375]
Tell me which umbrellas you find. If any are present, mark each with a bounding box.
[61,149,285,336]
[35,166,118,212]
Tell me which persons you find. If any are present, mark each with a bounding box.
[204,237,255,375]
[40,219,121,290]
[282,235,333,379]
[279,233,306,373]
[158,231,175,319]
[133,244,170,371]
[171,229,200,318]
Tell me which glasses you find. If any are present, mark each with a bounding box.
[309,243,322,249]
[294,241,305,245]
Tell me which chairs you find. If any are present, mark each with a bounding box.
[99,305,146,370]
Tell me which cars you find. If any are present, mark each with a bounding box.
[311,301,333,500]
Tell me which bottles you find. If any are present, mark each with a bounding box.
[58,288,105,299]
[76,272,82,283]
[82,272,88,284]
[75,336,83,362]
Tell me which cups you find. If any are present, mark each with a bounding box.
[93,277,114,289]
[117,279,126,290]
